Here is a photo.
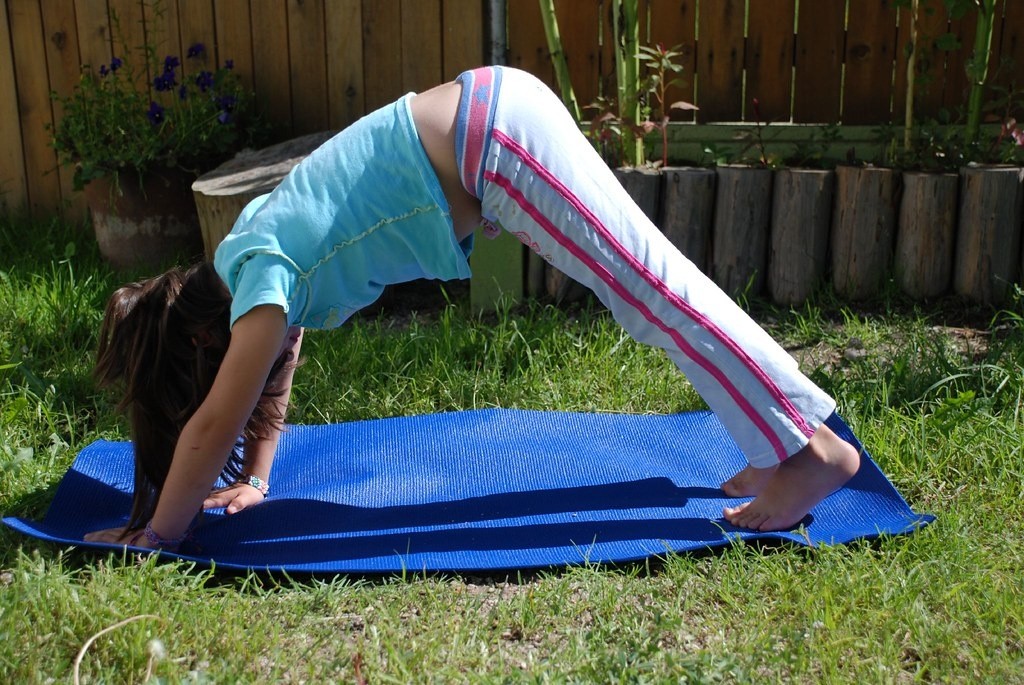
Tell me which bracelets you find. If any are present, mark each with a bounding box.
[143,521,194,551]
[235,470,270,495]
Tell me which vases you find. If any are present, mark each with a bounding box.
[85,171,196,267]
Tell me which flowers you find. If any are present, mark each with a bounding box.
[44,0,256,212]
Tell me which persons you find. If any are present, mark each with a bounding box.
[85,65,861,573]
